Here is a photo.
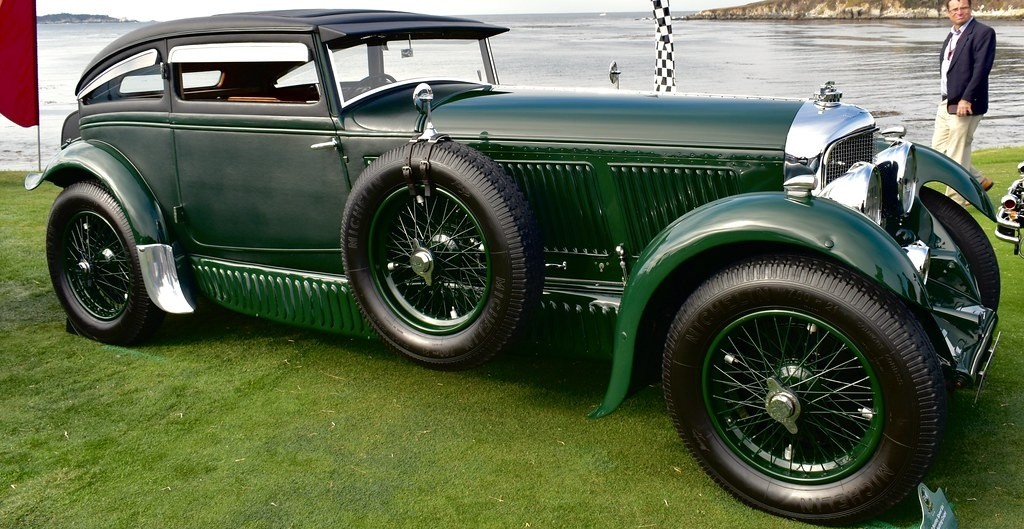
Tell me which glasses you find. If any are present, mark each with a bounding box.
[950,6,970,13]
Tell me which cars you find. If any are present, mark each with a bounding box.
[26,8,1003,525]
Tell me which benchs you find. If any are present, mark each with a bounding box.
[136,86,276,100]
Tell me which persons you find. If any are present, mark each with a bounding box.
[931,0,996,208]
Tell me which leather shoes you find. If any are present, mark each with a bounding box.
[965,179,994,207]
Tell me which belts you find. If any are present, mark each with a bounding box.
[942,96,947,100]
